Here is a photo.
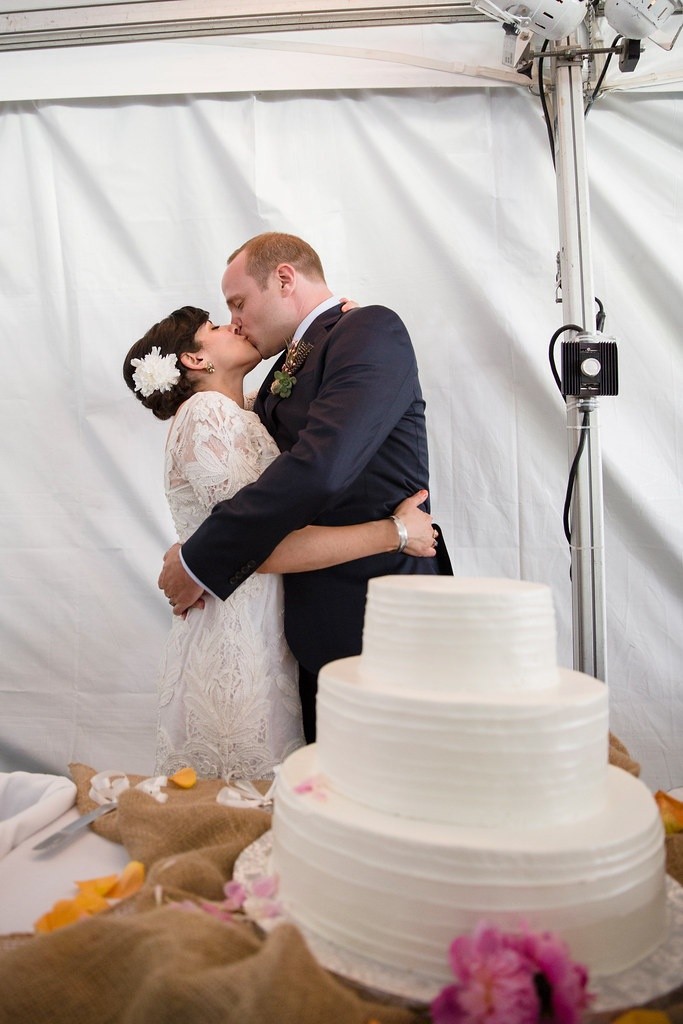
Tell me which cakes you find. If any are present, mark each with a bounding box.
[271,576,666,979]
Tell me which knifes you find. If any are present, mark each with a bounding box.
[32,802,123,851]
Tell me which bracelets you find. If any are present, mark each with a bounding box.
[390,516,407,554]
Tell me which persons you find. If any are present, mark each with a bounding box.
[122,234,455,782]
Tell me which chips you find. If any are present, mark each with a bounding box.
[170,767,197,790]
[36,862,144,933]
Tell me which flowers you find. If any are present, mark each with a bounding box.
[130,346,181,401]
[427,920,596,1024]
[270,330,315,398]
[34,859,149,935]
